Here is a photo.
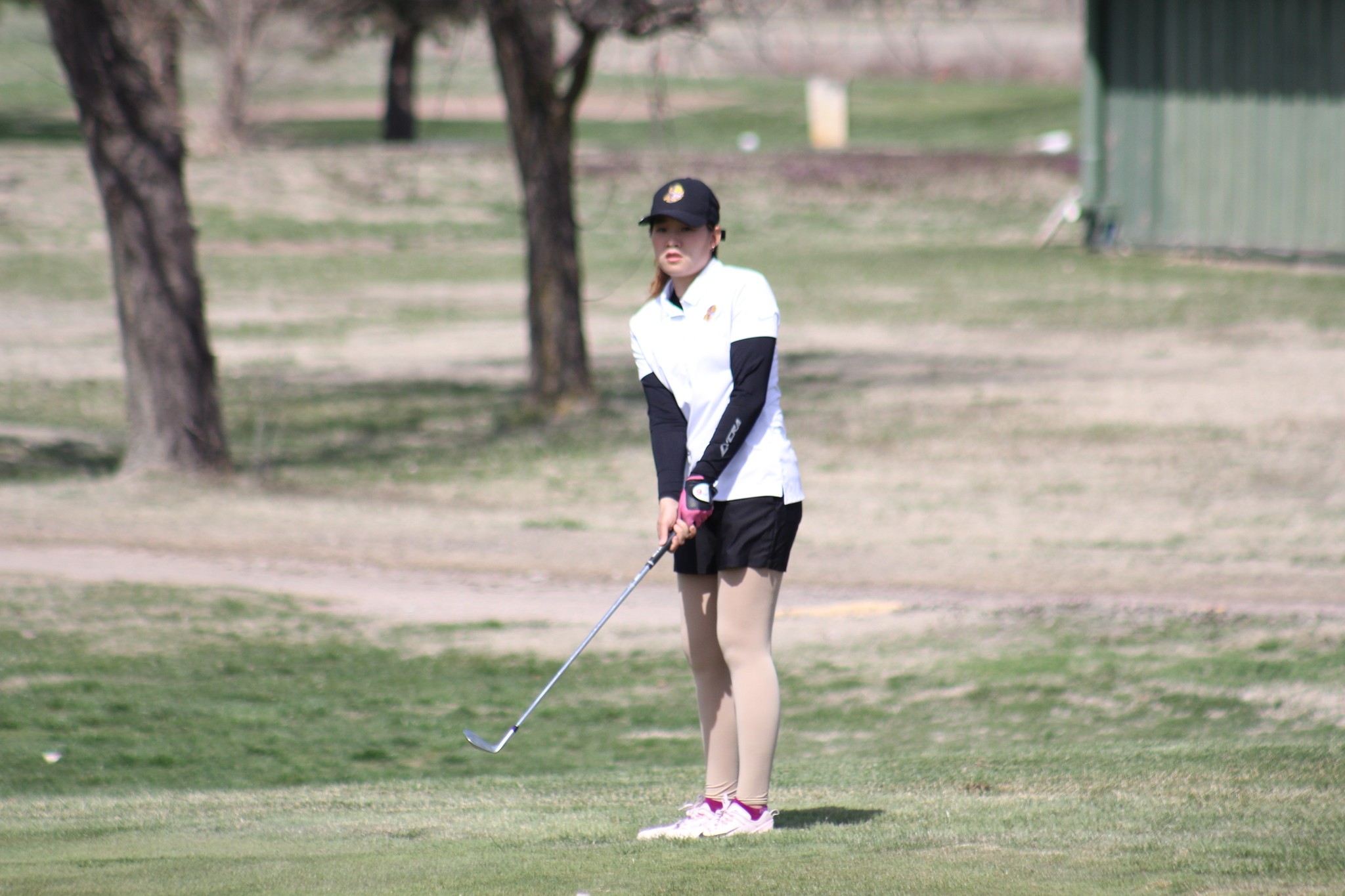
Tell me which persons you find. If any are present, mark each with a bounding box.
[629,178,804,841]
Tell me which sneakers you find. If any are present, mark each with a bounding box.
[688,800,777,838]
[637,795,731,842]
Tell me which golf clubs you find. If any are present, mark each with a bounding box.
[462,528,677,756]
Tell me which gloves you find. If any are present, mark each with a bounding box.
[676,475,714,529]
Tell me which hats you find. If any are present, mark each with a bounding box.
[638,178,720,230]
